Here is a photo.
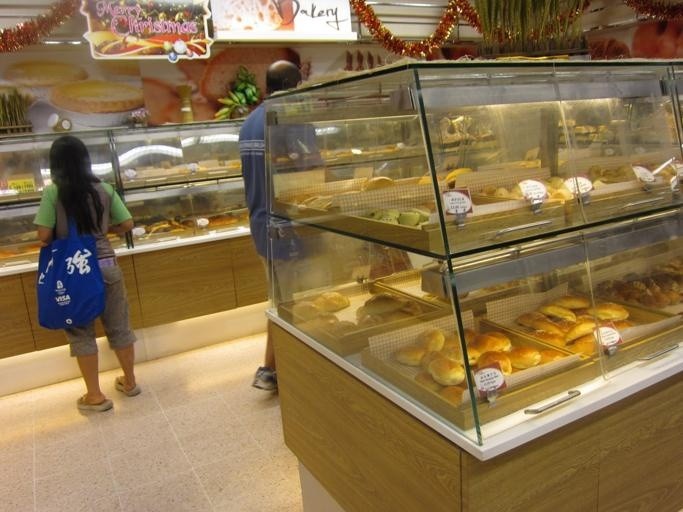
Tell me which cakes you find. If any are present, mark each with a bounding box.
[365,205,440,230]
[483,178,595,208]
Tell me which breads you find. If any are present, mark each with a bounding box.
[446,168,472,182]
[539,349,564,364]
[426,350,465,385]
[536,304,576,323]
[365,293,411,316]
[288,301,321,323]
[315,290,350,313]
[402,300,425,317]
[418,176,439,189]
[565,321,597,345]
[507,347,541,368]
[415,373,440,391]
[553,295,590,309]
[439,386,465,407]
[365,176,394,191]
[597,264,683,311]
[477,351,512,376]
[515,312,566,338]
[396,346,425,366]
[324,321,358,339]
[360,311,385,332]
[483,332,511,351]
[304,313,339,331]
[446,329,476,349]
[461,335,501,365]
[576,335,596,355]
[419,329,445,351]
[587,302,628,321]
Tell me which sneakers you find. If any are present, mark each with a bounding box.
[251,366,277,391]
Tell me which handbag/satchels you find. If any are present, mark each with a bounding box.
[35,216,106,331]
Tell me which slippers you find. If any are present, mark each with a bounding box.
[76,393,113,412]
[114,376,142,397]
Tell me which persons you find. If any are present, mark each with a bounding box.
[239,60,322,391]
[32,137,141,411]
